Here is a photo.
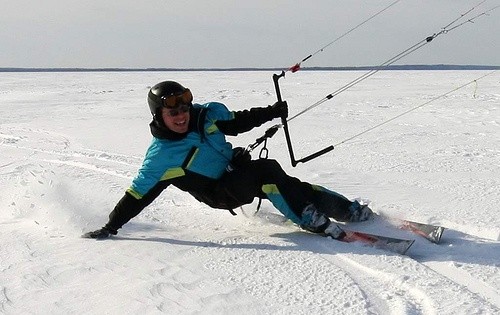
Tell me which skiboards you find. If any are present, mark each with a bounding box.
[347,214,445,245]
[252,209,415,255]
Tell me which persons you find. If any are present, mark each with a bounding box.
[81,81,378,244]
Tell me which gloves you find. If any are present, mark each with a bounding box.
[80,224,118,240]
[269,101,289,118]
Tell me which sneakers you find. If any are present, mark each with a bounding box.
[297,203,332,234]
[341,201,373,222]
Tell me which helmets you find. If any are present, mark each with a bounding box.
[147,81,193,117]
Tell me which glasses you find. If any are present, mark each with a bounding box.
[148,87,193,108]
[160,105,190,116]
